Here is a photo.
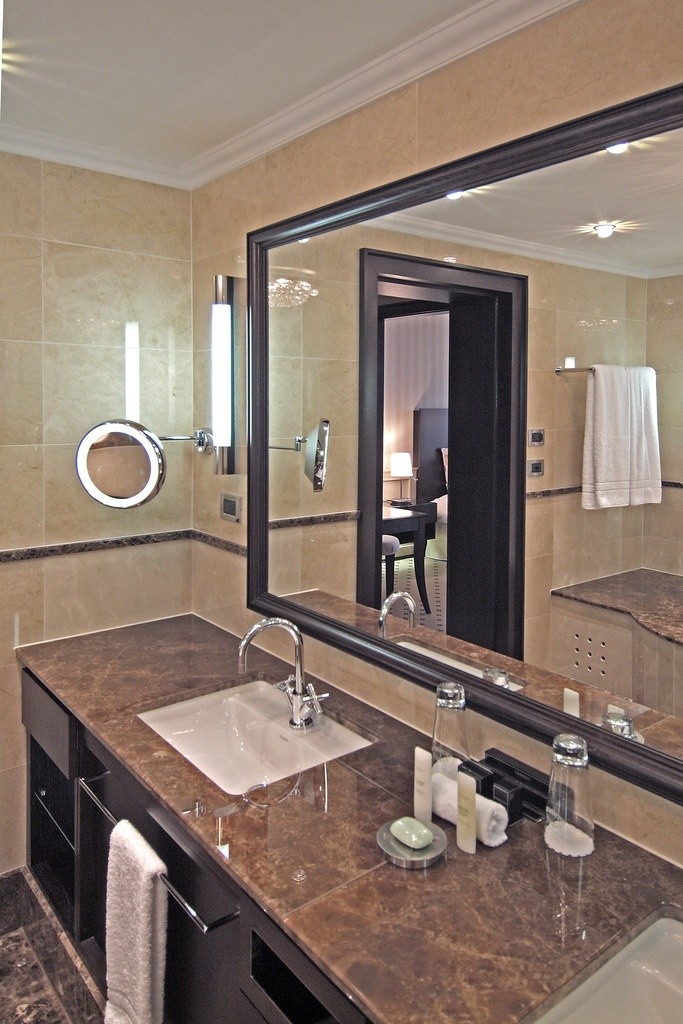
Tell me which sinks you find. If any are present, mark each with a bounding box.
[386,632,531,699]
[512,902,683,1023]
[134,672,385,799]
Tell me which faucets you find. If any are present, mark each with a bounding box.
[377,591,419,647]
[234,615,321,731]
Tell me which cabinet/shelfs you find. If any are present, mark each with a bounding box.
[15,614,683,1024]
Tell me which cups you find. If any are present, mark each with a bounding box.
[481,666,513,692]
[427,681,475,766]
[599,712,636,740]
[542,732,597,860]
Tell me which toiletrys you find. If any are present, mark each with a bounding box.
[412,744,433,823]
[562,688,583,720]
[454,770,478,855]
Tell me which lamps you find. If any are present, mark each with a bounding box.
[207,274,236,477]
[75,418,168,510]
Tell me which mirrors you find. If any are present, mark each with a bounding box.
[85,431,153,500]
[244,80,683,807]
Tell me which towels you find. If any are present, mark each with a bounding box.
[625,366,664,508]
[95,818,172,1022]
[433,771,510,848]
[580,364,631,511]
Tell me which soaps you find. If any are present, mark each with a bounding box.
[390,815,434,851]
